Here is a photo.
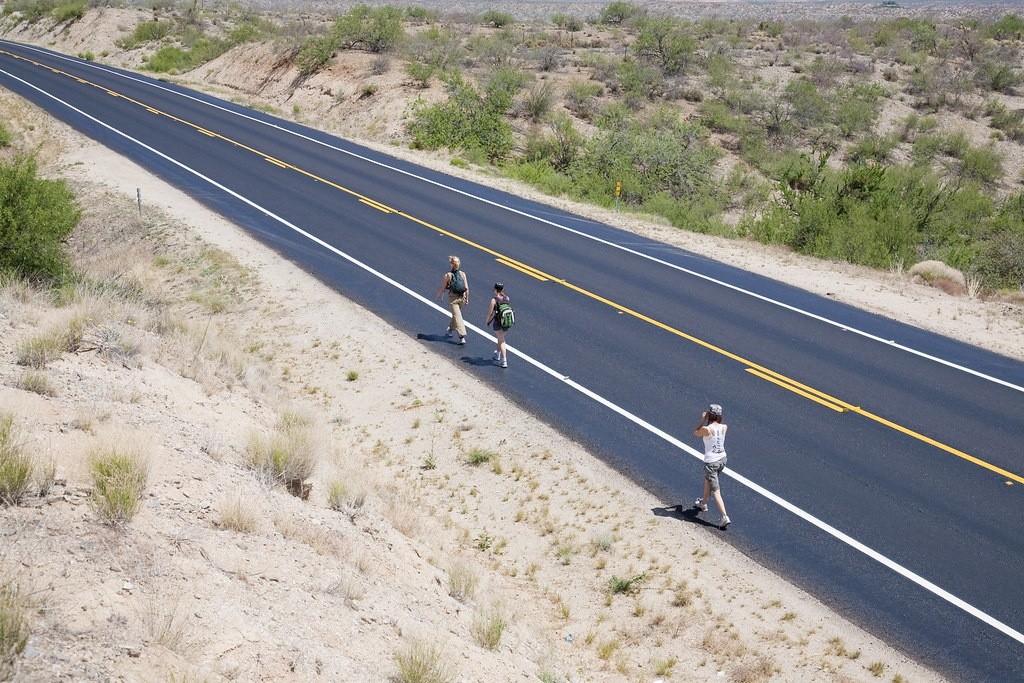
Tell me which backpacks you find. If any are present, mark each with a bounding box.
[494,296,515,328]
[449,271,467,293]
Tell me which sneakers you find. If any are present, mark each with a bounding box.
[457,337,466,344]
[718,514,731,530]
[493,359,507,368]
[446,326,453,336]
[494,349,500,361]
[694,497,708,512]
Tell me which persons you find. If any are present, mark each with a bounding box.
[486,282,507,368]
[695,404,731,529]
[436,256,470,345]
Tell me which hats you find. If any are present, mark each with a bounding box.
[706,404,722,416]
[494,282,504,289]
